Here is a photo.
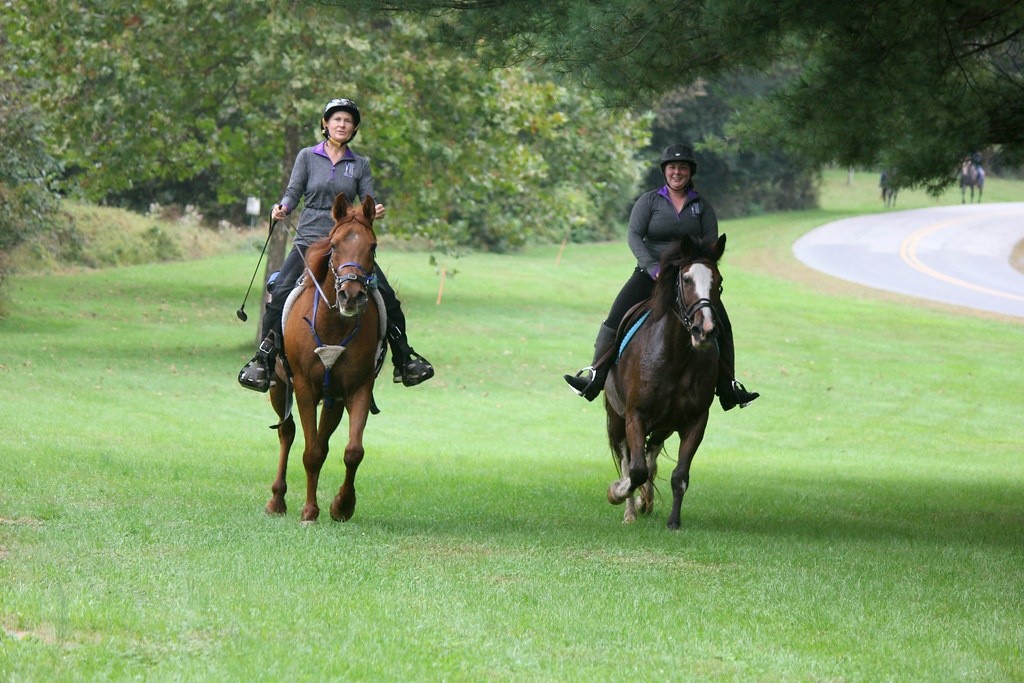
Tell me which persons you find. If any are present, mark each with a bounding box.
[238,96,435,392]
[881,150,985,192]
[564,142,761,412]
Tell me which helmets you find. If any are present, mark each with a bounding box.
[320,98,361,133]
[659,144,697,175]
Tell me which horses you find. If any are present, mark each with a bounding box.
[960,157,985,204]
[267,193,389,524]
[879,167,900,207]
[604,233,727,531]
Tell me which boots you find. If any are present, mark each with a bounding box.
[562,320,621,402]
[716,333,760,411]
[238,302,285,392]
[381,297,433,386]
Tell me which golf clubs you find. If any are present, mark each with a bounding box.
[237,205,283,322]
